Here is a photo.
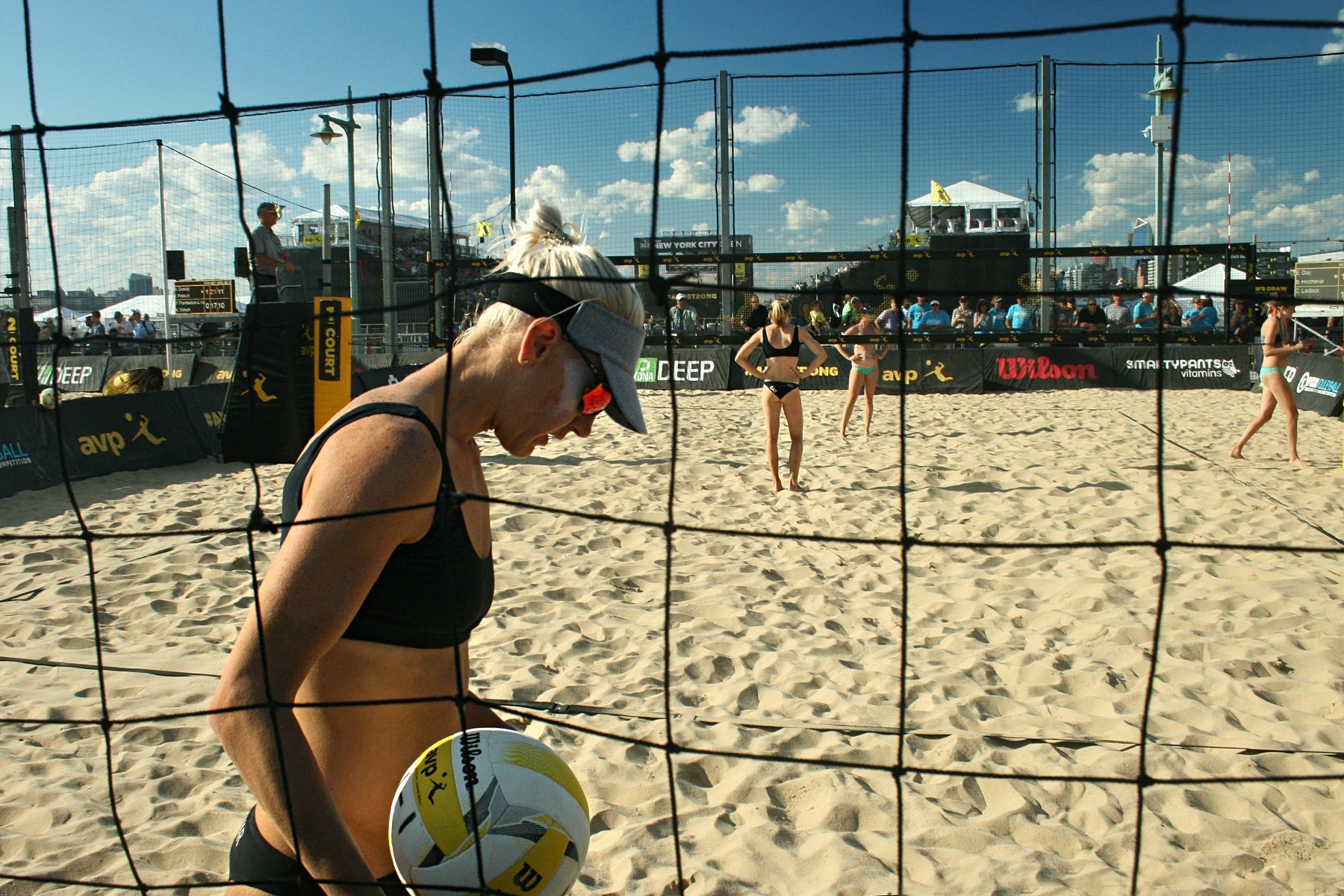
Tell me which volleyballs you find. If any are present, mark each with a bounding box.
[39,387,63,410]
[387,727,591,896]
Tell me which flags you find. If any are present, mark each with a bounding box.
[476,220,494,237]
[355,209,362,229]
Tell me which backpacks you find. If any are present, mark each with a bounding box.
[142,320,157,336]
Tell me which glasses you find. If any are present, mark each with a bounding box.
[1195,301,1201,303]
[1170,307,1176,309]
[1236,302,1243,305]
[1088,303,1096,306]
[132,313,138,316]
[560,332,613,416]
[751,301,759,304]
[266,210,277,215]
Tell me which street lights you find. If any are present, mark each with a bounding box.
[469,41,516,247]
[308,84,365,356]
[1147,33,1189,312]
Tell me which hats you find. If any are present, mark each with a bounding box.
[993,298,1003,302]
[492,271,650,435]
[43,318,54,322]
[131,308,140,314]
[959,295,968,301]
[676,293,687,300]
[930,300,940,305]
[850,297,860,304]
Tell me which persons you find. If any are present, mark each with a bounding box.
[1229,299,1313,466]
[645,290,1344,359]
[734,298,827,491]
[101,367,165,396]
[42,308,240,356]
[396,245,494,332]
[208,201,649,896]
[248,203,293,303]
[834,305,890,440]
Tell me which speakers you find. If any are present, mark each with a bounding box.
[166,251,185,279]
[234,247,250,278]
